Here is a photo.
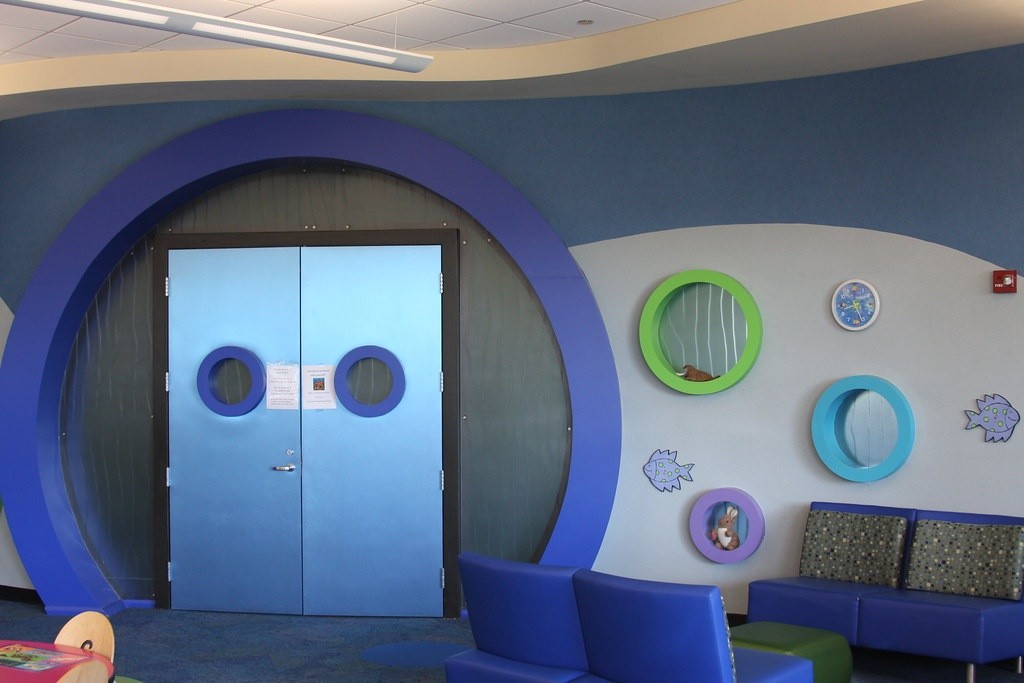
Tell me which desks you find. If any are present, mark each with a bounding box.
[728,618,854,682]
[1,639,116,683]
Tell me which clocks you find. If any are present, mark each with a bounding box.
[832,279,880,331]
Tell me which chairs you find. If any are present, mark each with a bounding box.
[53,611,116,662]
[59,660,110,682]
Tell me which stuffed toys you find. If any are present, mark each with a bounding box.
[676,365,720,383]
[710,506,741,550]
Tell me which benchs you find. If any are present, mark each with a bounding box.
[747,504,1023,683]
[443,554,816,683]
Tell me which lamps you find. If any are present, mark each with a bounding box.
[0,0,433,74]
